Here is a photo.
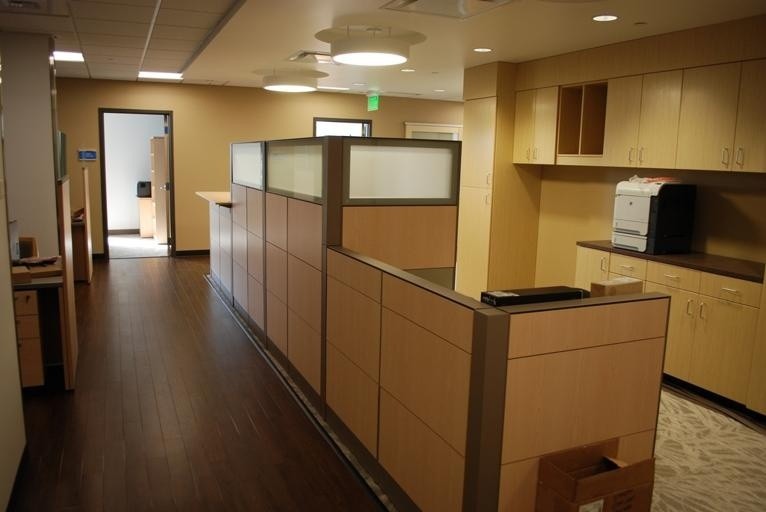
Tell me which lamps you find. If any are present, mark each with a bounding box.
[262,67,318,94]
[331,25,409,68]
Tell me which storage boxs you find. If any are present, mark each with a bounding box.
[534,436,655,512]
[590,275,643,298]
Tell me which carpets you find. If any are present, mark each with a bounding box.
[108,232,170,258]
[651,384,766,512]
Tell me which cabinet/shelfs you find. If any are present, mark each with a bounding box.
[453,63,543,302]
[602,69,683,170]
[746,267,766,429]
[136,194,152,238]
[609,251,648,293]
[574,246,610,292]
[12,275,66,387]
[512,85,562,166]
[676,58,766,173]
[150,136,167,244]
[644,261,761,406]
[556,79,608,166]
